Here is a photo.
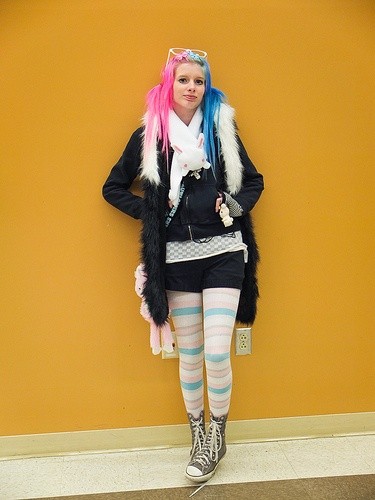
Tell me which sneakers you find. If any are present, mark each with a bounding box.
[184,412,228,482]
[187,410,206,461]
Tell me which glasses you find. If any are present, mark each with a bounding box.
[166,47,208,66]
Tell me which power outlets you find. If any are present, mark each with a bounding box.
[236,328,251,354]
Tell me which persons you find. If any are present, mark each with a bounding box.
[102,48,265,483]
